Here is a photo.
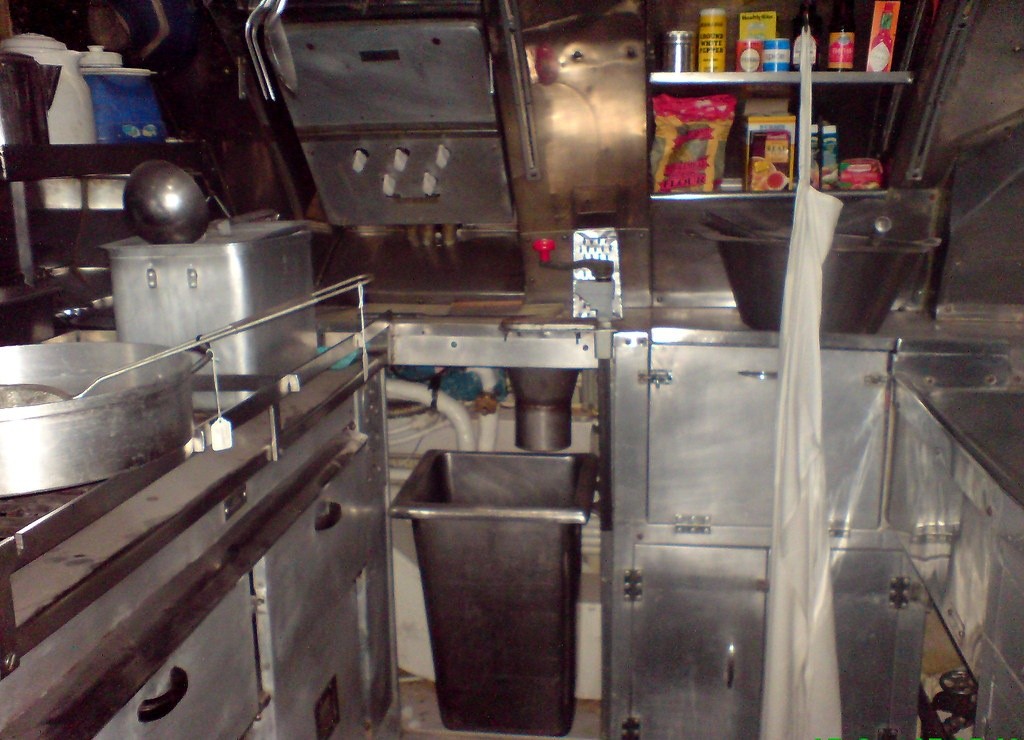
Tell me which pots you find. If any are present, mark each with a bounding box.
[686,227,942,334]
[0,341,210,498]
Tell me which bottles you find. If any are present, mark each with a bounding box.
[791,0,821,72]
[825,0,856,71]
[698,8,726,71]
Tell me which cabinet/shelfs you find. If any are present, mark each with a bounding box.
[0,358,403,740]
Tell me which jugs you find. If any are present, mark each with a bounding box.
[0,32,97,144]
[0,51,61,145]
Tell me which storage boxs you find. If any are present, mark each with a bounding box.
[743,95,796,192]
[813,121,884,192]
[866,0,901,73]
